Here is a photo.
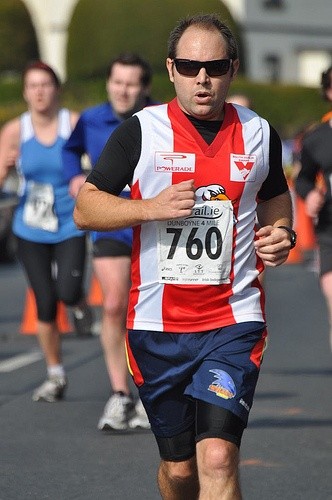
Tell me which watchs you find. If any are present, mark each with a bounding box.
[277,226,296,249]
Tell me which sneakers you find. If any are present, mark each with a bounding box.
[128,398,152,430]
[31,375,67,403]
[97,392,135,431]
[73,296,95,336]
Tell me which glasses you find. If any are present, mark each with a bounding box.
[171,57,233,78]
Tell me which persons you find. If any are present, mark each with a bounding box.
[0,61,95,402]
[224,65,332,329]
[62,54,153,432]
[73,11,297,500]
